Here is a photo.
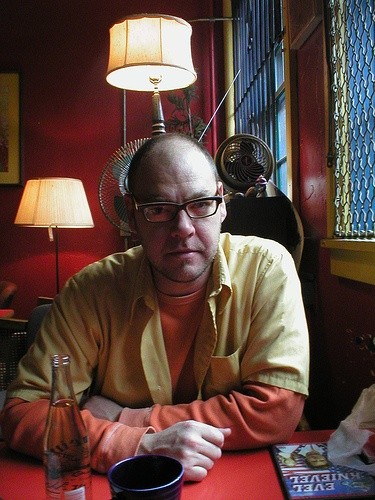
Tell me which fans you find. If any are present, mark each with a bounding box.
[215,133,274,191]
[98,137,149,236]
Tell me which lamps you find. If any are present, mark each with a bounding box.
[106,14,198,135]
[13,176,94,296]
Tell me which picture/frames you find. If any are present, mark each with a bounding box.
[1,73,22,187]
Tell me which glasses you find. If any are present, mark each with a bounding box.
[132,195,224,224]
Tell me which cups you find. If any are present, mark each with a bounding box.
[108,454,184,500]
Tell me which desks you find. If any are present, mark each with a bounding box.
[1,431,374,499]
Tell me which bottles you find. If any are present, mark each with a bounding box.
[42,353,91,500]
